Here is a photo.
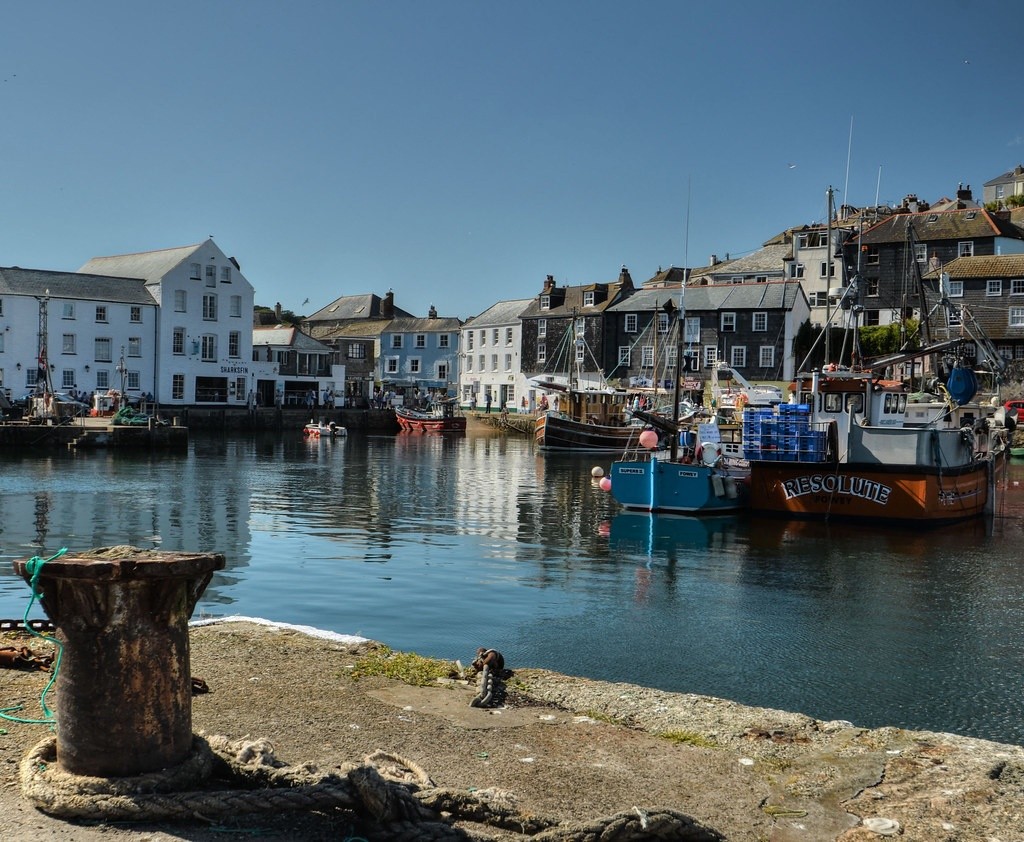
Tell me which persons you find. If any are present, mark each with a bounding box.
[540,393,547,411]
[554,396,559,410]
[323,389,335,410]
[376,390,393,409]
[142,392,153,400]
[634,396,640,408]
[485,393,492,413]
[520,396,525,414]
[72,384,95,402]
[627,394,633,407]
[425,391,432,403]
[248,389,254,407]
[306,389,316,412]
[417,389,423,407]
[470,394,475,412]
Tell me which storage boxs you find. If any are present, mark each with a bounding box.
[740,404,827,461]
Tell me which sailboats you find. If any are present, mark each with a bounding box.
[610,172,750,513]
[528,305,702,458]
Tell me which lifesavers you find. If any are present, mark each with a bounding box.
[696,442,721,468]
[451,423,459,429]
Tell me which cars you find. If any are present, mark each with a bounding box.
[1003,400,1024,423]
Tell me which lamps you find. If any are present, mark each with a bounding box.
[50,363,55,372]
[17,362,21,370]
[84,363,90,373]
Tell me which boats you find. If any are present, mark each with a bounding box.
[740,110,1013,528]
[304,417,347,438]
[393,392,469,435]
